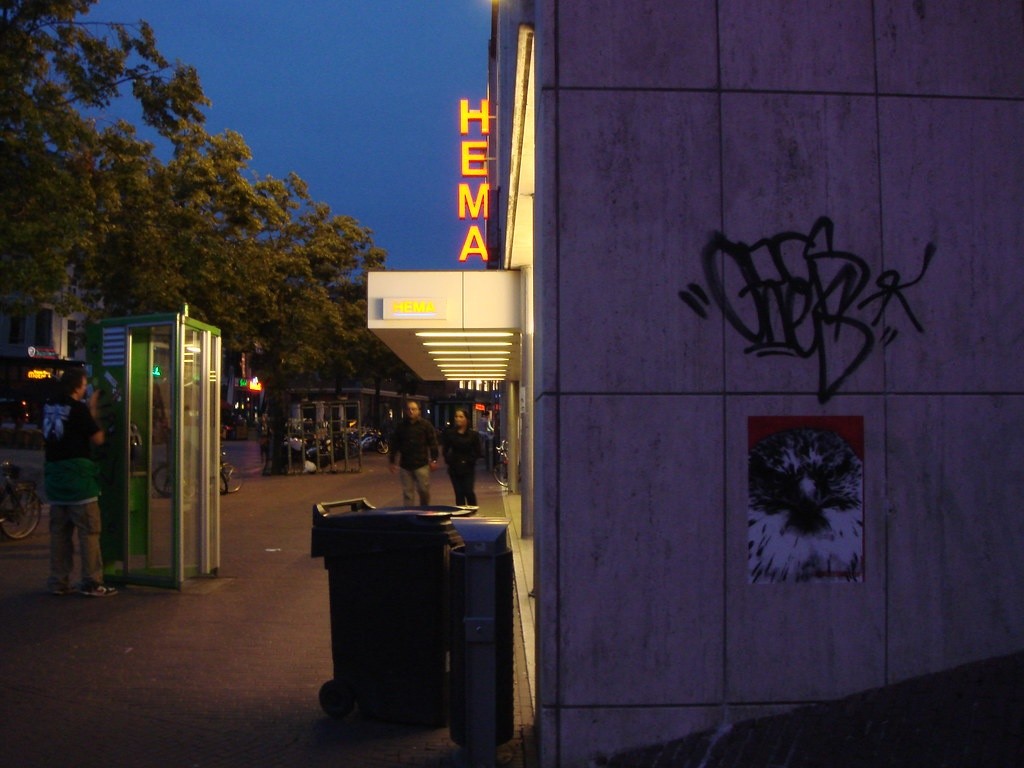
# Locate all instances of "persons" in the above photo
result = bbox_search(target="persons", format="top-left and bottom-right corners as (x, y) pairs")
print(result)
(43, 366), (119, 596)
(442, 407), (484, 512)
(388, 400), (440, 507)
(258, 414), (273, 464)
(480, 410), (495, 433)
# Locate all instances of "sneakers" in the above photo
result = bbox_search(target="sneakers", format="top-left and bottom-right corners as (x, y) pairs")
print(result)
(79, 583), (119, 597)
(51, 585), (77, 595)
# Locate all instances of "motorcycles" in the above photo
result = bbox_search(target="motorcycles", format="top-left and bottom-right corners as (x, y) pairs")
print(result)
(285, 421), (389, 464)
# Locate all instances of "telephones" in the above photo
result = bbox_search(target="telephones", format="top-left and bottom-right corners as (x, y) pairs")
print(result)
(130, 423), (143, 447)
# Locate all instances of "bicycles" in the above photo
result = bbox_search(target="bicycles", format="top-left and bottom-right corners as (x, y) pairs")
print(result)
(493, 439), (510, 487)
(153, 449), (244, 498)
(0, 460), (42, 541)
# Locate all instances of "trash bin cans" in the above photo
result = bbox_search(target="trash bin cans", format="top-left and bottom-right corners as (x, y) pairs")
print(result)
(312, 497), (480, 718)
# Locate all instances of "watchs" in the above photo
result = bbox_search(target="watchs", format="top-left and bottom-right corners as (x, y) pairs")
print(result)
(433, 460), (436, 463)
